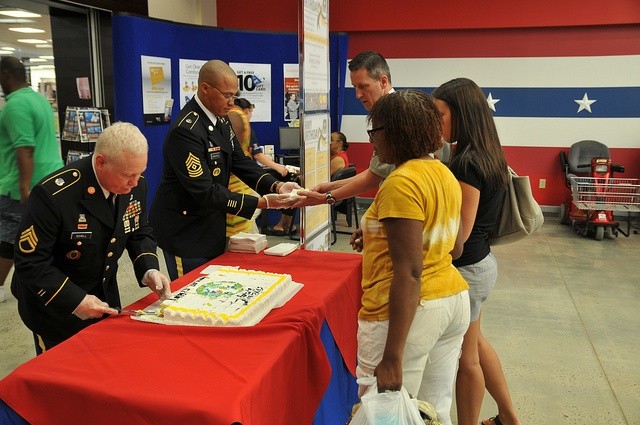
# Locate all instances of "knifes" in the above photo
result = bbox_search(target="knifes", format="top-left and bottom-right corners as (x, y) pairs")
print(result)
(112, 306), (157, 314)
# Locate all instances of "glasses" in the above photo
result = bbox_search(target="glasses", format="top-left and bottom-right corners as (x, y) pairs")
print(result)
(367, 126), (384, 141)
(207, 81), (240, 100)
(331, 137), (340, 142)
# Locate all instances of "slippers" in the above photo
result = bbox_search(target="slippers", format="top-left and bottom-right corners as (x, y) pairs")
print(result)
(262, 224), (285, 235)
(284, 224), (297, 235)
(481, 413), (504, 424)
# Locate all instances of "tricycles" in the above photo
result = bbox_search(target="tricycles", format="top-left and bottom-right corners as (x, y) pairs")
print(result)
(559, 140), (640, 241)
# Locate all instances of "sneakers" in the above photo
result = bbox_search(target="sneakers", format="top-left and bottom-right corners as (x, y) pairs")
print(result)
(0, 285), (6, 303)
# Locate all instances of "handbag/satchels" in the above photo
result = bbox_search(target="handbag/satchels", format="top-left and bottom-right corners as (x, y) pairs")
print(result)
(345, 395), (441, 425)
(487, 163), (546, 246)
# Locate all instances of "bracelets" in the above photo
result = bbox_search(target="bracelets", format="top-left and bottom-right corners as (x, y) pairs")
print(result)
(272, 180), (280, 193)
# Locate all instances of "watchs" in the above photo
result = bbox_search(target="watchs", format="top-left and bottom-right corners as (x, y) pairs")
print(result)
(324, 190), (336, 207)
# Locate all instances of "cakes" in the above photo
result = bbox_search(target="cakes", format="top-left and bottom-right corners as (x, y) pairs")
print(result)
(159, 267), (292, 326)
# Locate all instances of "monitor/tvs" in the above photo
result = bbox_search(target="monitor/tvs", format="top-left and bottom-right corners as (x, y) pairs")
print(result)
(278, 127), (300, 156)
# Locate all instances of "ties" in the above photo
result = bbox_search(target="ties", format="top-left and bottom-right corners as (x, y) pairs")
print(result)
(106, 191), (116, 220)
(215, 117), (222, 136)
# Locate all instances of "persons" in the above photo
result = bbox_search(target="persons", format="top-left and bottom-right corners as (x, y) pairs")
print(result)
(234, 96), (289, 177)
(0, 54), (64, 304)
(328, 131), (349, 176)
(430, 81), (526, 425)
(10, 123), (172, 356)
(290, 50), (452, 208)
(225, 107), (258, 240)
(286, 94), (300, 119)
(148, 60), (306, 284)
(351, 92), (470, 425)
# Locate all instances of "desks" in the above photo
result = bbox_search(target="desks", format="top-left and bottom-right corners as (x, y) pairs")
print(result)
(0, 244), (364, 425)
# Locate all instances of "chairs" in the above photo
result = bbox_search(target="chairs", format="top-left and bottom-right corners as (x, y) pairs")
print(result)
(565, 140), (610, 183)
(289, 194), (337, 244)
(332, 167), (359, 245)
